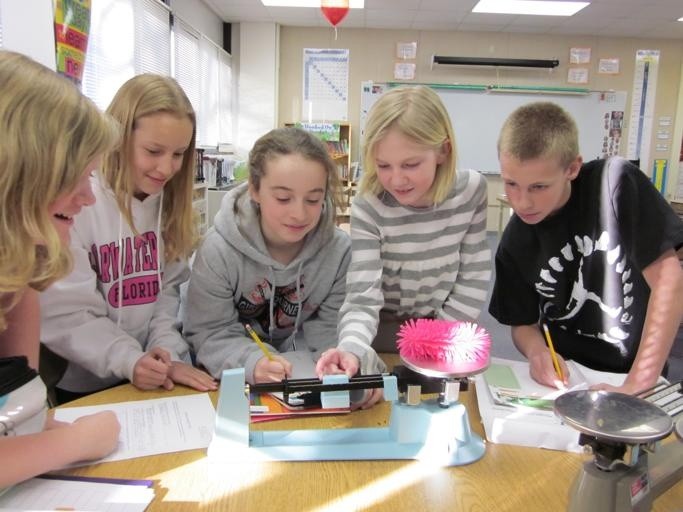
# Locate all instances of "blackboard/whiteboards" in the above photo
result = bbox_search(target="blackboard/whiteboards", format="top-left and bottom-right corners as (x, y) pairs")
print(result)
(360, 81), (628, 176)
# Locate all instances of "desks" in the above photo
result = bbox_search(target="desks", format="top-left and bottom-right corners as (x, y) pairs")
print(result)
(39, 352), (683, 512)
(495, 194), (514, 237)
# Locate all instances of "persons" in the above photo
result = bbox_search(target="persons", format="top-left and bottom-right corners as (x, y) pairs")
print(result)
(488, 102), (682, 394)
(39, 72), (219, 390)
(182, 127), (380, 410)
(316, 83), (493, 379)
(1, 50), (120, 489)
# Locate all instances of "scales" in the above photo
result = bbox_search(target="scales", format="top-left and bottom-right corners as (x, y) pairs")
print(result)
(553, 388), (683, 512)
(206, 318), (494, 470)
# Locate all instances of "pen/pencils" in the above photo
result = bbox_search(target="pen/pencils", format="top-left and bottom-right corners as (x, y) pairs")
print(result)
(543, 323), (563, 380)
(245, 323), (274, 361)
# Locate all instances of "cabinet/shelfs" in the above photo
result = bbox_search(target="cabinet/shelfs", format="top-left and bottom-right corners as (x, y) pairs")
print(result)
(284, 121), (352, 205)
(192, 148), (212, 235)
(205, 154), (240, 226)
